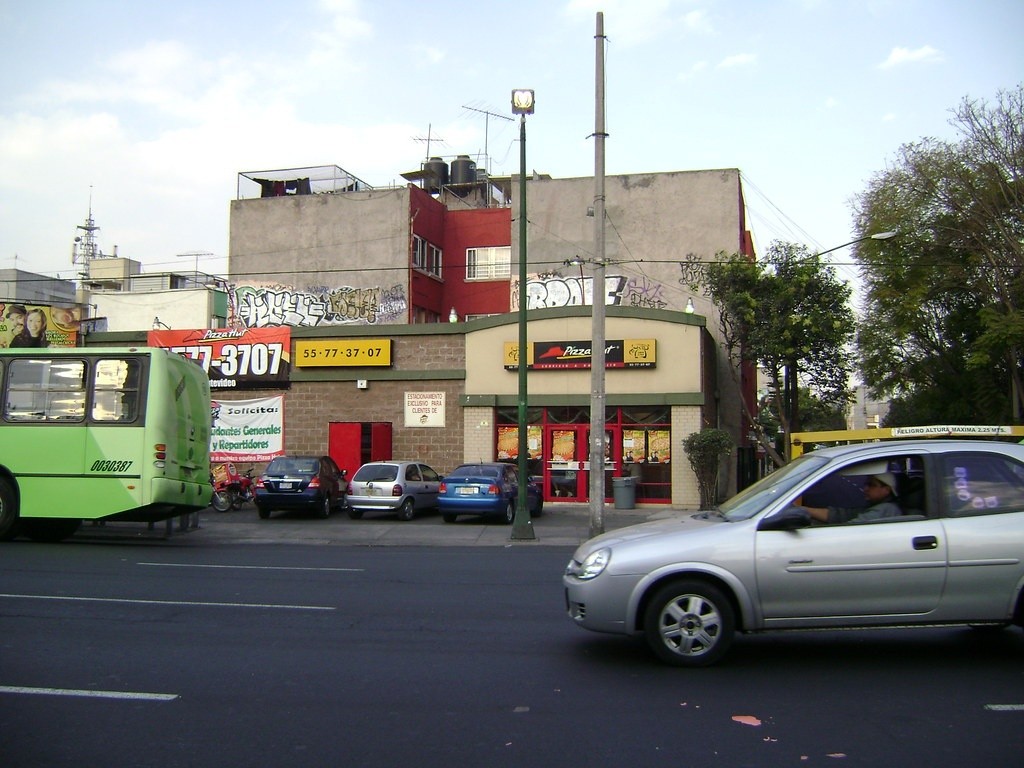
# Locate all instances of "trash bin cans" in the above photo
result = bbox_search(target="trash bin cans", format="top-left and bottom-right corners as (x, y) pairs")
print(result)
(612, 477), (637, 509)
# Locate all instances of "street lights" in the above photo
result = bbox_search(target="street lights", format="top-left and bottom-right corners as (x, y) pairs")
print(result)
(510, 87), (538, 540)
(782, 231), (900, 422)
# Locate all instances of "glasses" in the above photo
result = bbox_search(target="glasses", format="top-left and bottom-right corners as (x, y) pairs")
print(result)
(864, 480), (882, 488)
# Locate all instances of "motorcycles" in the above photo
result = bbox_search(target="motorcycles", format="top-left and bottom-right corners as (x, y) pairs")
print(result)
(226, 466), (259, 511)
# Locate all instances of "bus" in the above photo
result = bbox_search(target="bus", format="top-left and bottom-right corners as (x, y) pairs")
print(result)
(0, 345), (213, 539)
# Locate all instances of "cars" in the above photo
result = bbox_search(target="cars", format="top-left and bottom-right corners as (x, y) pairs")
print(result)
(252, 455), (350, 519)
(340, 459), (443, 520)
(437, 460), (544, 523)
(562, 437), (1024, 668)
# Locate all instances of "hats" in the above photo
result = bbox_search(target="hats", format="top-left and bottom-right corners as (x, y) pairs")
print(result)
(874, 472), (897, 497)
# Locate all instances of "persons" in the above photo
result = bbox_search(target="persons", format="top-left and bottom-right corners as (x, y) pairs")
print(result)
(792, 470), (902, 523)
(626, 451), (633, 461)
(5, 304), (48, 348)
(651, 451), (659, 462)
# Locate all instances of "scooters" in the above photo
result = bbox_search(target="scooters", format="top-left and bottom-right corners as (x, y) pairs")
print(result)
(207, 459), (233, 513)
